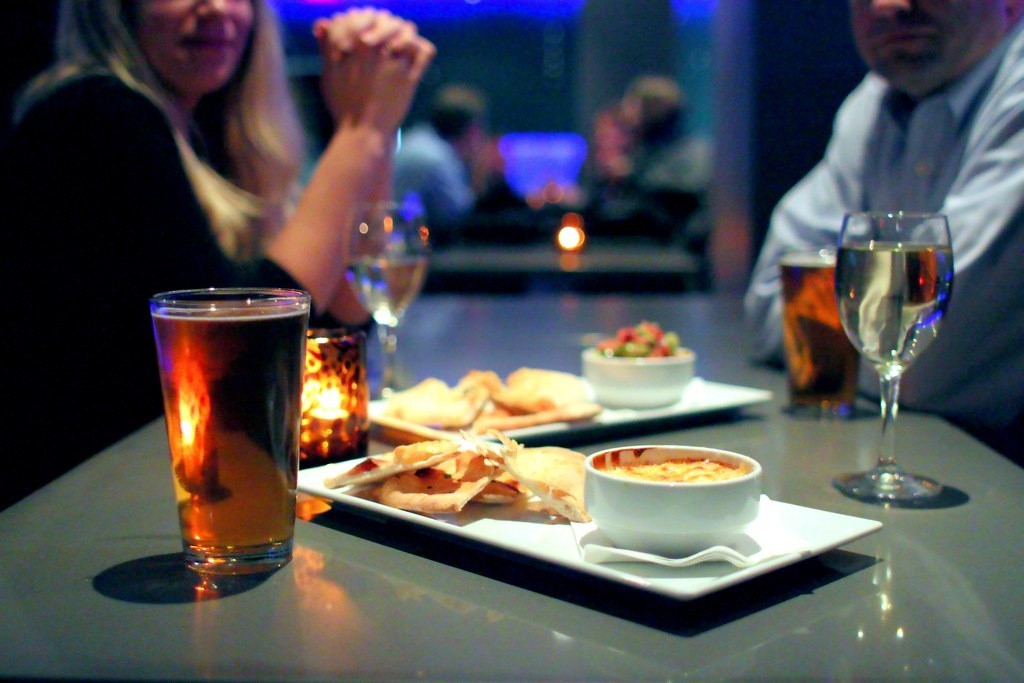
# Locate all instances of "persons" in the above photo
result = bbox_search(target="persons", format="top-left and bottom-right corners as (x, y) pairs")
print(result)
(425, 77), (715, 245)
(746, 1), (1024, 433)
(0, 0), (435, 503)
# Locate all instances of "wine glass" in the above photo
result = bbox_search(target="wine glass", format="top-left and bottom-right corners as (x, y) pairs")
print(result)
(342, 199), (427, 401)
(833, 211), (954, 502)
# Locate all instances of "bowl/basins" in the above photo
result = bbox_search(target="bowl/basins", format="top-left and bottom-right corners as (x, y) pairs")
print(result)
(583, 348), (696, 410)
(583, 446), (763, 555)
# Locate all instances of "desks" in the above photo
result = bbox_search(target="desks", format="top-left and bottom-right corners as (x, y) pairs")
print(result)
(0, 296), (1024, 683)
(436, 240), (706, 295)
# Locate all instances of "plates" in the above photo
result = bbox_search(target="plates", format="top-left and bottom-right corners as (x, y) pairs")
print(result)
(366, 378), (774, 442)
(297, 443), (884, 600)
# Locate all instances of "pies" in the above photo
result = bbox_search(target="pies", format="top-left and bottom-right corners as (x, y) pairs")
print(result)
(324, 367), (601, 521)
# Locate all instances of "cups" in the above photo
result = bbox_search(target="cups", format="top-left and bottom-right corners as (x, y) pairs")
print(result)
(296, 324), (370, 464)
(151, 287), (312, 576)
(778, 247), (861, 417)
